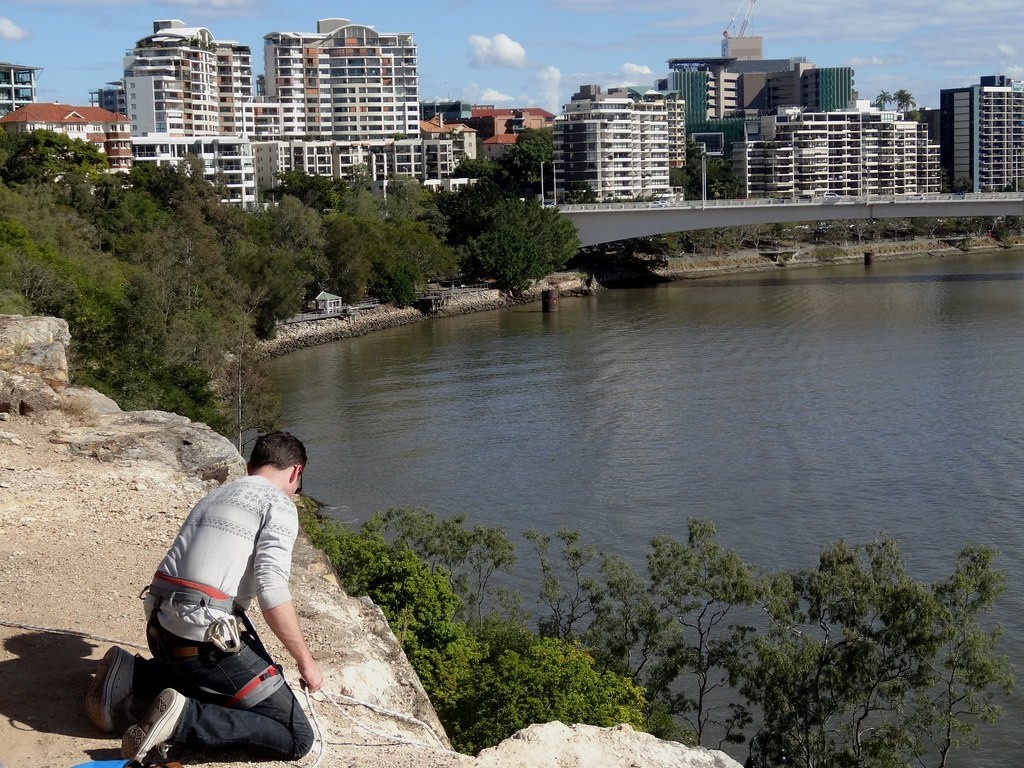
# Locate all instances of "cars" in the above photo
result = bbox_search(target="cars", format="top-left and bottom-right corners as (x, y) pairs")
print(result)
(949, 192), (966, 200)
(906, 192), (925, 201)
(767, 196), (793, 205)
(647, 200), (670, 207)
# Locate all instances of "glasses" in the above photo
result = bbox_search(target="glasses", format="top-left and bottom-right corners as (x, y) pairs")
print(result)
(292, 465), (302, 494)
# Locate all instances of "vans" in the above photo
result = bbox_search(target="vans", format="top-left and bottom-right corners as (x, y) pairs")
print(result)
(824, 193), (842, 199)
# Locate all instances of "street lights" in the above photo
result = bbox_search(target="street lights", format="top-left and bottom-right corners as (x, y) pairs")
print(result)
(552, 162), (557, 207)
(541, 161), (545, 206)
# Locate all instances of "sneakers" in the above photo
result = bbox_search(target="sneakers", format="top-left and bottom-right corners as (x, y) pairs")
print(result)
(84, 645), (134, 734)
(120, 687), (189, 764)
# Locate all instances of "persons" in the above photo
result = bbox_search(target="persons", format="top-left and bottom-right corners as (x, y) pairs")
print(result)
(84, 432), (323, 766)
